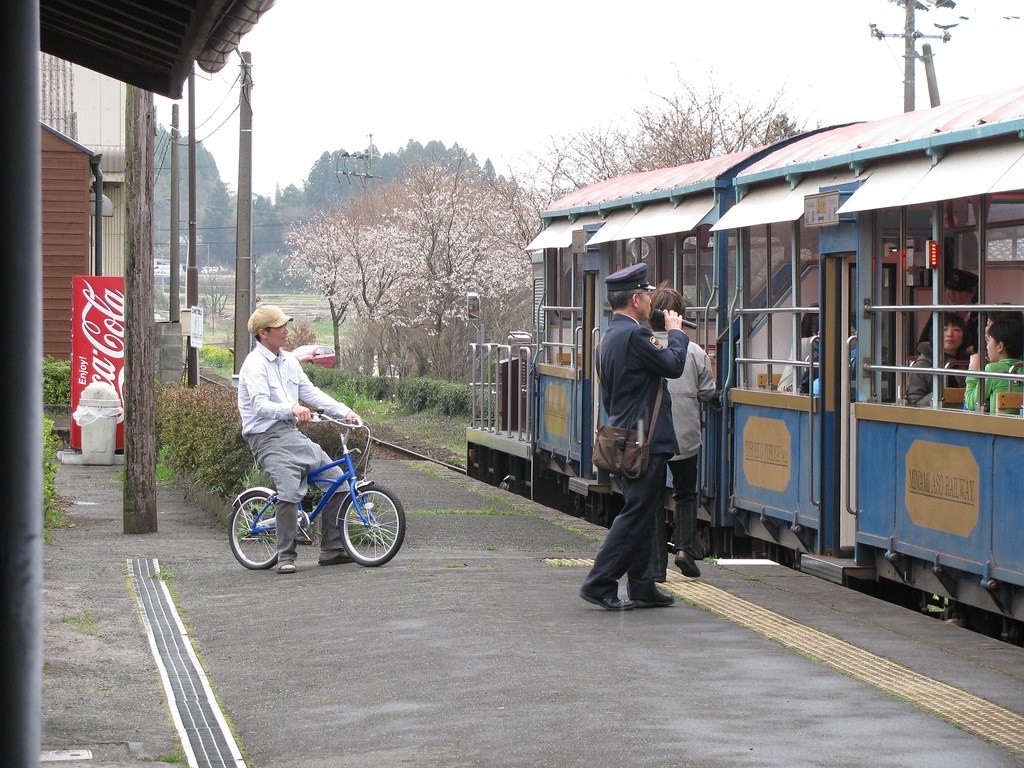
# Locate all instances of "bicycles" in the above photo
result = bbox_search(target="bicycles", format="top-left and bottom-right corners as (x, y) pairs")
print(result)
(225, 407), (407, 569)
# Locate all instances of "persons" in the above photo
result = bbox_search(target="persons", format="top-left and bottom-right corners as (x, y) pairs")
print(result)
(578, 262), (690, 611)
(800, 314), (819, 393)
(776, 303), (819, 394)
(906, 313), (970, 408)
(985, 303), (1024, 335)
(850, 319), (858, 380)
(647, 279), (718, 584)
(916, 268), (988, 388)
(237, 305), (364, 574)
(964, 320), (1024, 415)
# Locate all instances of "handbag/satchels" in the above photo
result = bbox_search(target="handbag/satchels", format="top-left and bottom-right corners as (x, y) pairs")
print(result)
(592, 426), (650, 478)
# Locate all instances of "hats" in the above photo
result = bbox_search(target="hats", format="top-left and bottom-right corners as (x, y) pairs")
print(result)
(944, 267), (978, 291)
(247, 306), (293, 337)
(603, 263), (658, 292)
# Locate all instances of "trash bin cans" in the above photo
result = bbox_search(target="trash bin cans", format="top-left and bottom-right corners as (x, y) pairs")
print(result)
(71, 381), (124, 466)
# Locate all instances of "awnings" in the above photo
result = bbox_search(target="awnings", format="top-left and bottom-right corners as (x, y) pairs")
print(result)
(584, 192), (715, 245)
(834, 139), (1024, 213)
(524, 213), (602, 250)
(709, 169), (855, 232)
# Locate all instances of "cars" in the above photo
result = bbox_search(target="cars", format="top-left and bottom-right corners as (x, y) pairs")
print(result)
(291, 344), (336, 368)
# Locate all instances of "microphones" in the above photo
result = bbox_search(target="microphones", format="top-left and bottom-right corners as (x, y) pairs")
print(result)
(653, 308), (698, 330)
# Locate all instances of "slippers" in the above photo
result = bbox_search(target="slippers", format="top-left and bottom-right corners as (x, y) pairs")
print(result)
(318, 551), (356, 565)
(275, 560), (296, 574)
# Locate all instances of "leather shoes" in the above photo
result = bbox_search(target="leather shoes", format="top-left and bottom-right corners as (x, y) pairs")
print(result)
(654, 574), (666, 581)
(675, 551), (701, 578)
(633, 590), (675, 606)
(579, 589), (636, 610)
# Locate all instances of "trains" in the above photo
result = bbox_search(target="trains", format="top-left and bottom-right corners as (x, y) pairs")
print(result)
(466, 97), (1024, 645)
